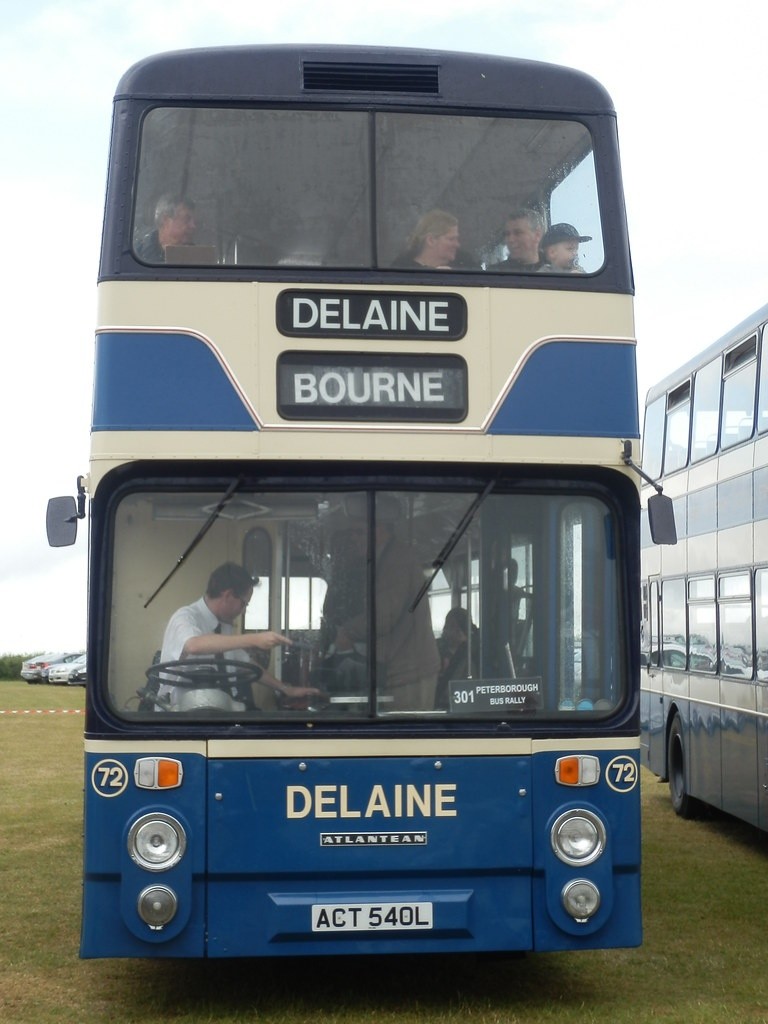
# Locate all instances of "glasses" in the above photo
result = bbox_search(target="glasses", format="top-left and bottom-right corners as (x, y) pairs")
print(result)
(234, 595), (249, 608)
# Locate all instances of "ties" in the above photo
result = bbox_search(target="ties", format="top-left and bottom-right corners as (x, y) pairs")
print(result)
(214, 623), (233, 698)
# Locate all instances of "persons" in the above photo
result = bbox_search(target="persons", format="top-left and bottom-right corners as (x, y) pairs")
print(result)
(439, 608), (473, 668)
(336, 517), (441, 711)
(543, 224), (594, 273)
(153, 563), (319, 712)
(490, 208), (550, 273)
(137, 194), (197, 263)
(394, 210), (461, 271)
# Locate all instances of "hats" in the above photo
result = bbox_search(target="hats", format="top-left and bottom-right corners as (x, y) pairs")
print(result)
(539, 223), (592, 247)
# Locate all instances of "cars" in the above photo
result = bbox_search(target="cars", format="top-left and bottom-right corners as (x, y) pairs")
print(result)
(20, 652), (69, 684)
(49, 652), (87, 685)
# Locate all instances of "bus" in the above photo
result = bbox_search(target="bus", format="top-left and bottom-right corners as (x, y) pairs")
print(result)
(640, 300), (767, 837)
(45, 42), (679, 962)
